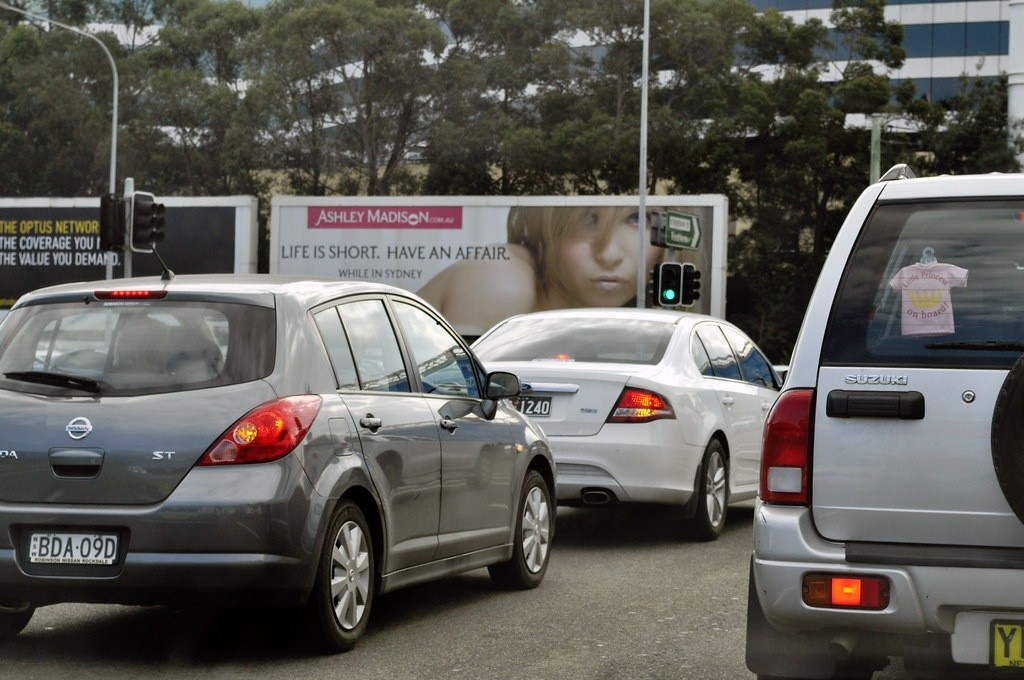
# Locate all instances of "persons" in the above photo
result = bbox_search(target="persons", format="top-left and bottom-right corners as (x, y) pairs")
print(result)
(414, 205), (708, 336)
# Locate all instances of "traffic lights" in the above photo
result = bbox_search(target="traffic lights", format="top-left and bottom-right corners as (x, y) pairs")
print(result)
(681, 263), (701, 307)
(100, 192), (126, 252)
(130, 191), (166, 253)
(658, 262), (681, 307)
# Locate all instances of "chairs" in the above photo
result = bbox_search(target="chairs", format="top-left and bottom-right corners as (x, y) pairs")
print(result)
(547, 339), (598, 358)
(166, 338), (224, 373)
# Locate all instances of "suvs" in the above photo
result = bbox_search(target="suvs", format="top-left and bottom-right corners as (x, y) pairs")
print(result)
(745, 162), (1024, 680)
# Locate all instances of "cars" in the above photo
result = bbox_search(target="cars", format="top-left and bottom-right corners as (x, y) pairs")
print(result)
(0, 274), (557, 650)
(467, 308), (788, 541)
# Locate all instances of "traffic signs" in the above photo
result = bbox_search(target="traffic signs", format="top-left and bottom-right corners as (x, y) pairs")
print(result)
(664, 211), (702, 250)
